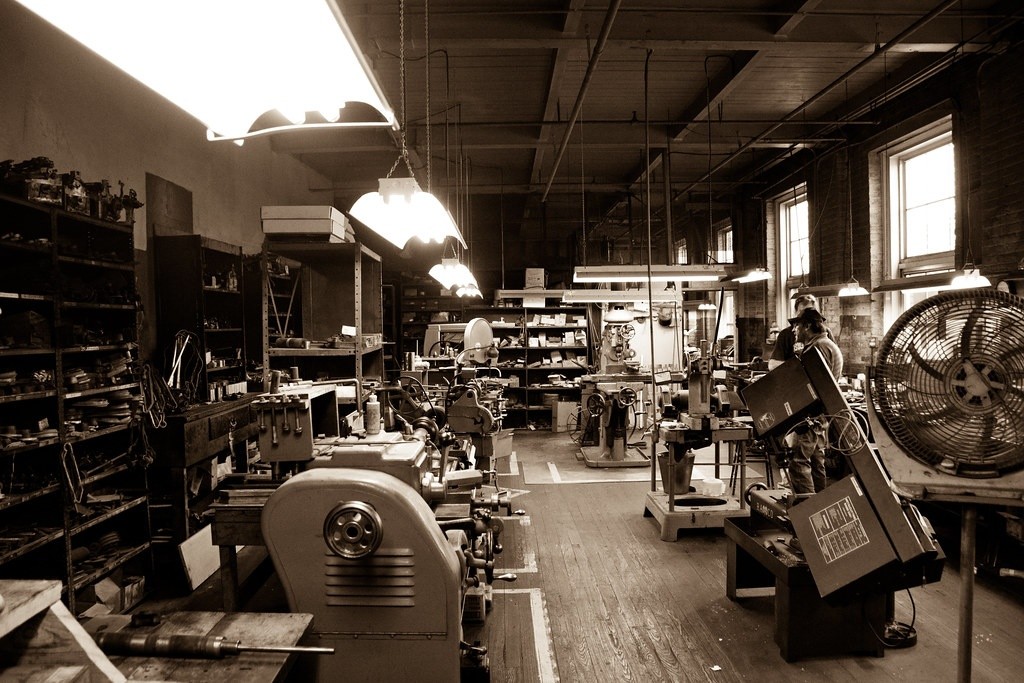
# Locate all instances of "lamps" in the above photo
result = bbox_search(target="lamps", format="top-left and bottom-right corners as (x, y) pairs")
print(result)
(15, 0), (484, 299)
(789, 78), (870, 299)
(871, 13), (992, 293)
(493, 107), (717, 305)
(717, 151), (774, 284)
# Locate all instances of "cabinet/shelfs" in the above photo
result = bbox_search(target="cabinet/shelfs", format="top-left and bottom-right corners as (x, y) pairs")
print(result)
(1, 159), (590, 623)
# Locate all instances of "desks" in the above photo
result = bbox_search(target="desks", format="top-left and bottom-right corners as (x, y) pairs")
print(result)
(145, 392), (270, 541)
(0, 579), (315, 682)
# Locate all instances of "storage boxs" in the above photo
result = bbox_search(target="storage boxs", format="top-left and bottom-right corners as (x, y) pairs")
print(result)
(75, 565), (146, 616)
(577, 356), (586, 367)
(552, 401), (579, 433)
(260, 206), (345, 227)
(540, 393), (559, 406)
(261, 220), (345, 241)
(548, 373), (566, 384)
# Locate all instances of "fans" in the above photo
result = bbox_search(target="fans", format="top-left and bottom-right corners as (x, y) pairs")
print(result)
(863, 287), (1023, 683)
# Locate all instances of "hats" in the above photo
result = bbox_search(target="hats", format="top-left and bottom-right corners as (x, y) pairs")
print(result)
(787, 307), (826, 323)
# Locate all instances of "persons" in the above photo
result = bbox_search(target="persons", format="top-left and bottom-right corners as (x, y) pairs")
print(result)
(769, 295), (836, 481)
(788, 308), (844, 505)
